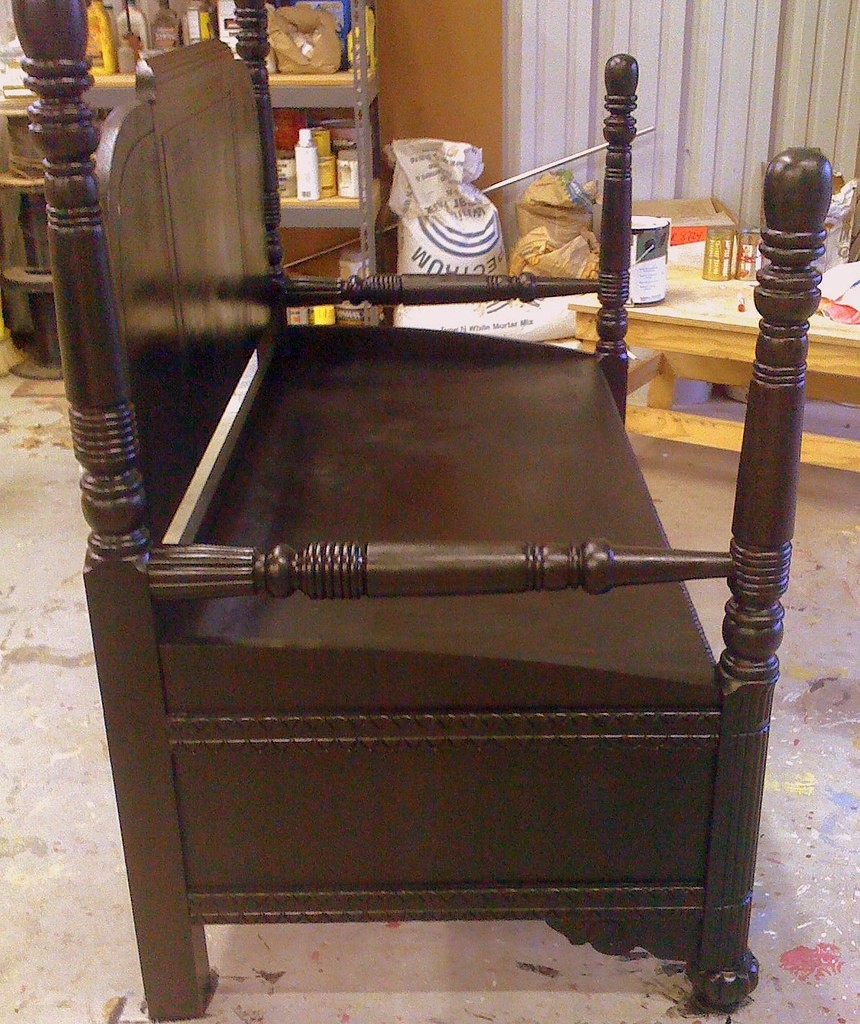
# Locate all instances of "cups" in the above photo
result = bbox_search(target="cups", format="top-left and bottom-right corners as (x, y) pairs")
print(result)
(735, 232), (764, 281)
(702, 225), (735, 281)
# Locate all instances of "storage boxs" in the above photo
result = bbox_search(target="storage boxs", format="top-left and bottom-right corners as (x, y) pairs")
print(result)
(631, 195), (739, 247)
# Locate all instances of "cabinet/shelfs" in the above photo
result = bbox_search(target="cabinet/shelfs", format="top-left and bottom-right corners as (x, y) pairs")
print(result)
(0, 0), (391, 327)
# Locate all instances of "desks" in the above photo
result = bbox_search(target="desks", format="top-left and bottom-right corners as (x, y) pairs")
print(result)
(568, 266), (860, 477)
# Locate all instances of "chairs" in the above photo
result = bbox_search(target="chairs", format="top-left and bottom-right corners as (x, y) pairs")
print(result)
(8, 0), (834, 1022)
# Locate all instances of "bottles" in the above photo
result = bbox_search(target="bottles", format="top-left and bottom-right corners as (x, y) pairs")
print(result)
(294, 128), (320, 201)
(336, 149), (359, 199)
(311, 129), (338, 199)
(275, 150), (297, 198)
(86, 0), (181, 76)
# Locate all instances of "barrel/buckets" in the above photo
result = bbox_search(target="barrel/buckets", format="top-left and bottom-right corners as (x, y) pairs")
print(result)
(628, 216), (671, 309)
